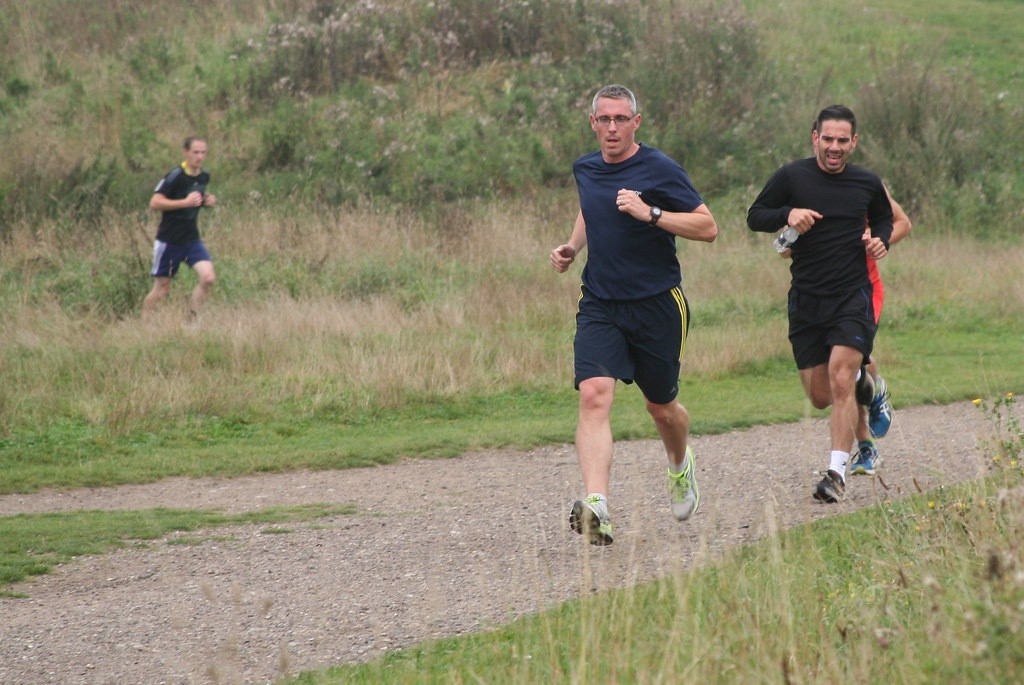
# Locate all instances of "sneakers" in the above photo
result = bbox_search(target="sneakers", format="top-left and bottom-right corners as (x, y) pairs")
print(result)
(569, 498), (613, 546)
(667, 444), (701, 522)
(849, 439), (882, 475)
(867, 375), (893, 439)
(855, 364), (875, 406)
(812, 470), (845, 503)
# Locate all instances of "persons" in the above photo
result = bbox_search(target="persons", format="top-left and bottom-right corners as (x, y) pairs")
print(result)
(544, 83), (722, 548)
(776, 115), (914, 479)
(742, 102), (898, 508)
(140, 133), (219, 330)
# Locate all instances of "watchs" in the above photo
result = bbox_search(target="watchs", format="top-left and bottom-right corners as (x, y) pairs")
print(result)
(646, 206), (662, 227)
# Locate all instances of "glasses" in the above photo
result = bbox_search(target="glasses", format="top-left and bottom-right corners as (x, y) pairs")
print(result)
(595, 114), (635, 126)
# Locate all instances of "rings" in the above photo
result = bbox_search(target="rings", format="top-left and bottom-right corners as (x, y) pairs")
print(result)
(619, 199), (623, 206)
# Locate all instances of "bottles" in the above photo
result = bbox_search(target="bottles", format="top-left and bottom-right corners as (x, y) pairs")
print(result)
(772, 226), (799, 254)
